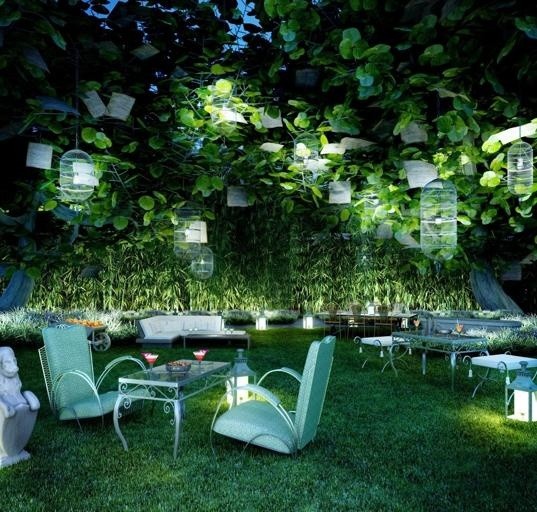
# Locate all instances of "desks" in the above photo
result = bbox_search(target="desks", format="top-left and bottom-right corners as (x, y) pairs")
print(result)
(111, 357), (232, 460)
(314, 310), (420, 337)
(391, 329), (488, 394)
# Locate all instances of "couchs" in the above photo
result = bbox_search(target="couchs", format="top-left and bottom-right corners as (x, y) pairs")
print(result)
(134, 309), (224, 350)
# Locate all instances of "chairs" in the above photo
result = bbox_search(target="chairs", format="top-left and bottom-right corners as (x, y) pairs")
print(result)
(34, 323), (156, 435)
(322, 298), (404, 342)
(207, 334), (337, 462)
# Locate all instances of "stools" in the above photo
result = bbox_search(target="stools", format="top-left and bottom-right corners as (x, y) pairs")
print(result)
(355, 334), (412, 379)
(461, 351), (537, 402)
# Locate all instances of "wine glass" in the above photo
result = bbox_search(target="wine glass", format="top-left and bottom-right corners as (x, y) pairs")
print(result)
(456, 324), (463, 338)
(414, 320), (420, 331)
(193, 350), (206, 369)
(144, 354), (158, 376)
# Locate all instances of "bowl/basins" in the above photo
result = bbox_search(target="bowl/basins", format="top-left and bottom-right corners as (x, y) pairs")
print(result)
(440, 330), (452, 334)
(165, 365), (191, 372)
